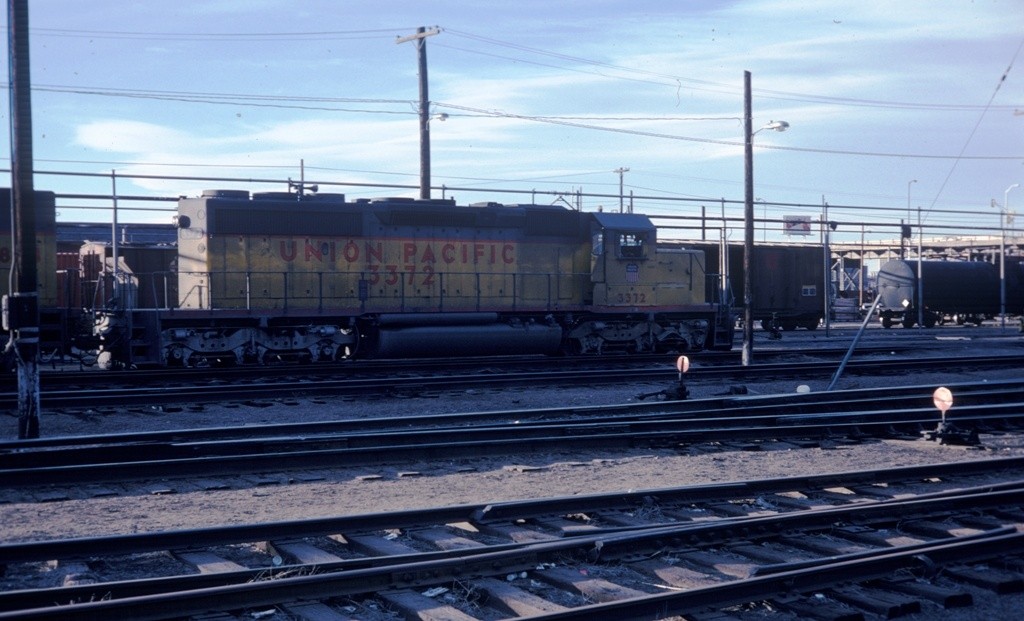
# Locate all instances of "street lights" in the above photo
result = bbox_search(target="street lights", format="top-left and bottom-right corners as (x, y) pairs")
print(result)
(1001, 181), (1024, 235)
(419, 111), (450, 201)
(753, 196), (768, 243)
(738, 69), (788, 369)
(907, 178), (919, 232)
(611, 165), (632, 212)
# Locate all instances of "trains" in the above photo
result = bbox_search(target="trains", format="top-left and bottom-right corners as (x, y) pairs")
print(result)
(874, 251), (1024, 329)
(659, 235), (834, 334)
(0, 182), (741, 387)
